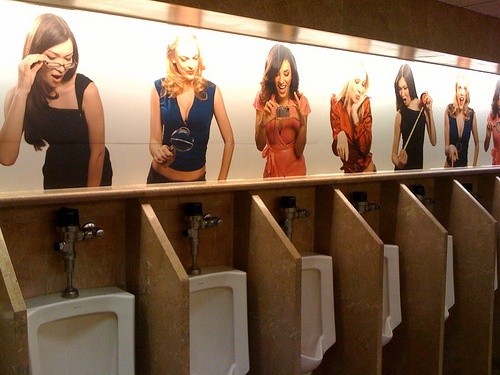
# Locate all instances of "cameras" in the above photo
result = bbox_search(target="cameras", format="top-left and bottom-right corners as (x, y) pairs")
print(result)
(276, 106), (289, 118)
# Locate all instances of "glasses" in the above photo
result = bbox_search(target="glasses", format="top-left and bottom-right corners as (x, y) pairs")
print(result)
(44, 60), (77, 70)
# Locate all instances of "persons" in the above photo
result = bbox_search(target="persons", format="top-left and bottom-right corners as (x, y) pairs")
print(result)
(484, 80), (500, 167)
(0, 14), (112, 190)
(253, 43), (311, 178)
(391, 64), (436, 171)
(147, 32), (235, 183)
(444, 78), (480, 167)
(329, 70), (377, 173)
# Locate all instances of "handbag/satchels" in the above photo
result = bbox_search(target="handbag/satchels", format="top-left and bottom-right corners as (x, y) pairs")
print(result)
(397, 105), (423, 170)
